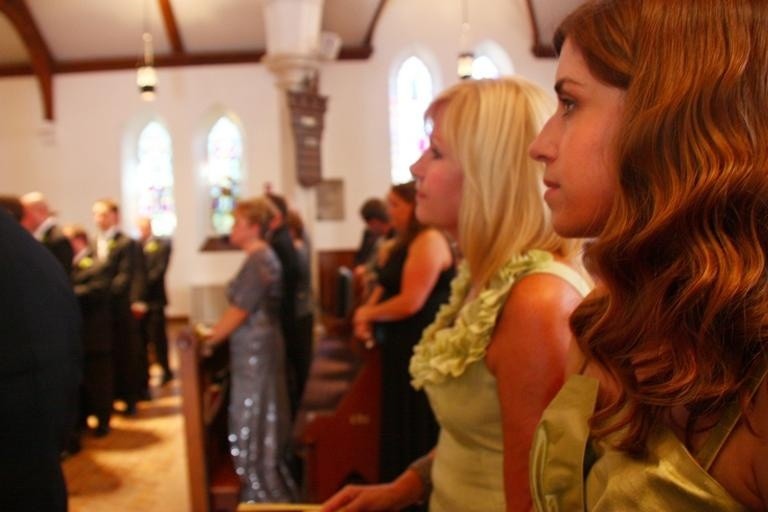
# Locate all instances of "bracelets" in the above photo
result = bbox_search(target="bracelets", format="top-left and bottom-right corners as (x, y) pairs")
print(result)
(408, 456), (433, 503)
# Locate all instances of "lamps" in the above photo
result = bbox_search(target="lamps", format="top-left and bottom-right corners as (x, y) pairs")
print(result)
(457, 1), (475, 81)
(136, 0), (158, 102)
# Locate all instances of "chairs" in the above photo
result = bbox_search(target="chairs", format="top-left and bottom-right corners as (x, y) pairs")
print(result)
(296, 267), (377, 504)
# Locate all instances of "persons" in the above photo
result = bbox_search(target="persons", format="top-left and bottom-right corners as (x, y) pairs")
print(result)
(207, 196), (297, 506)
(23, 189), (174, 456)
(318, 73), (589, 512)
(258, 192), (308, 422)
(288, 211), (313, 401)
(527, 0), (767, 512)
(1, 200), (89, 512)
(352, 179), (453, 479)
